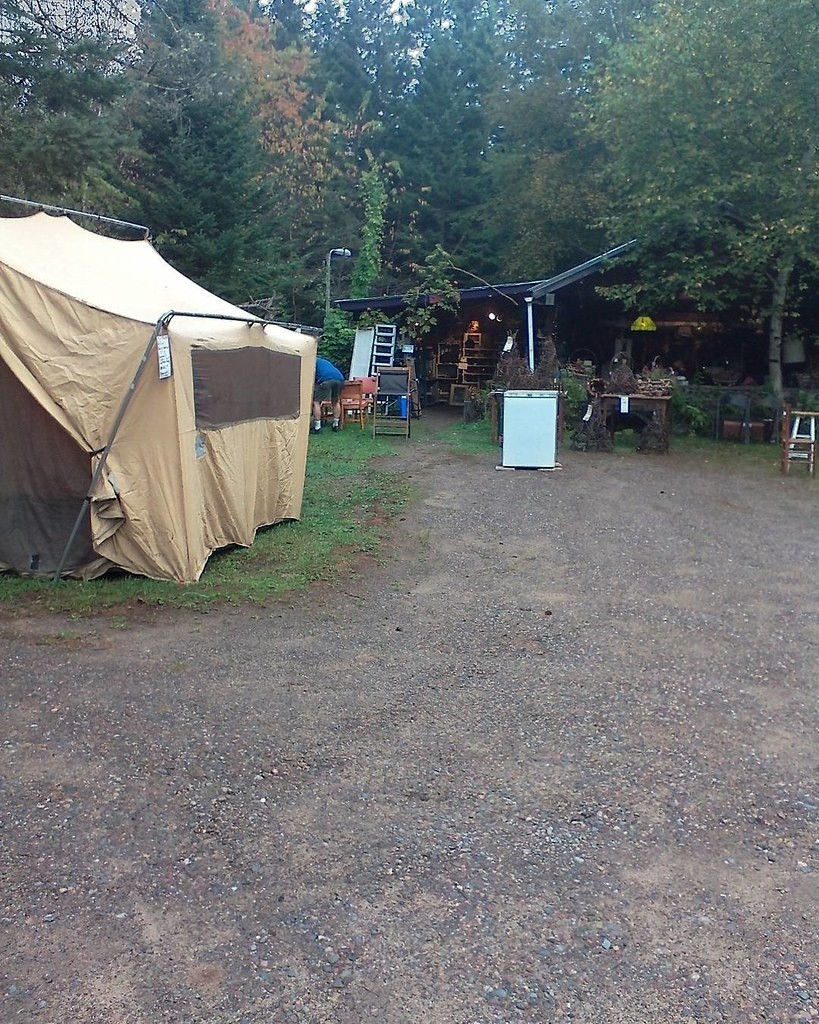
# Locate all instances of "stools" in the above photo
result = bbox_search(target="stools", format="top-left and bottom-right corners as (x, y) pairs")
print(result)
(784, 411), (819, 472)
(322, 376), (377, 428)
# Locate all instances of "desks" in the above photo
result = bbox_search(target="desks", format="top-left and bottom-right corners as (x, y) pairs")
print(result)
(599, 393), (672, 441)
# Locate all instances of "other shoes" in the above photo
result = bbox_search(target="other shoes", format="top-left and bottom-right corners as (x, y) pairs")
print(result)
(332, 426), (338, 432)
(309, 427), (322, 434)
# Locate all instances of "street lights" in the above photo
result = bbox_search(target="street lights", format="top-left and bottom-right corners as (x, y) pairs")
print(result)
(324, 245), (352, 317)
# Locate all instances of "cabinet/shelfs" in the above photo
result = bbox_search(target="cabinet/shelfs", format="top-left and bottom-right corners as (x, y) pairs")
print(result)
(419, 333), (501, 405)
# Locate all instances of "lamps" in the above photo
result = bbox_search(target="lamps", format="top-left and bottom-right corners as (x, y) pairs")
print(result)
(631, 316), (656, 331)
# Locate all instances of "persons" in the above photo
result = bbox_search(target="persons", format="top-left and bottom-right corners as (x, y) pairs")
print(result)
(310, 358), (345, 435)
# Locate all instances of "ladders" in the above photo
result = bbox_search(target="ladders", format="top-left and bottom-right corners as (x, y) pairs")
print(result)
(368, 324), (397, 415)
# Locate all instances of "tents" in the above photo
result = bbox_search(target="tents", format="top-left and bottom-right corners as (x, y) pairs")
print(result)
(0, 195), (324, 586)
